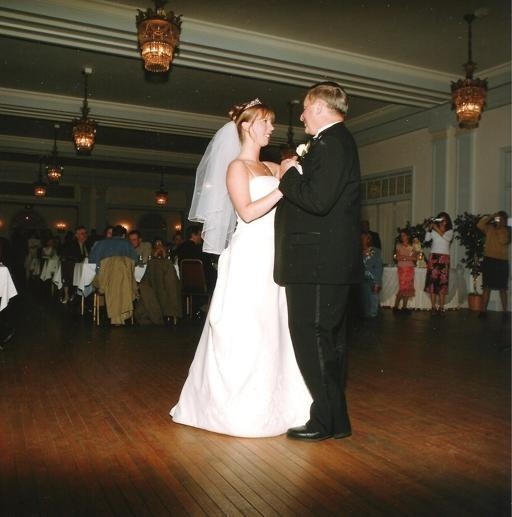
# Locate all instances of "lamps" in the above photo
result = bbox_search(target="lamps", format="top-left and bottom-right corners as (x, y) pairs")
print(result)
(134, 1), (186, 74)
(34, 156), (47, 198)
(74, 66), (100, 155)
(155, 165), (170, 203)
(45, 128), (64, 179)
(447, 13), (487, 129)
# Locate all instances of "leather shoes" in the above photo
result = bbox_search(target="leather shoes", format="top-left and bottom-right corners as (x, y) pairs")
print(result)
(285, 418), (353, 442)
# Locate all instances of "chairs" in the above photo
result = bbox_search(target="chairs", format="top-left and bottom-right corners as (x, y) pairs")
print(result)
(24, 232), (218, 329)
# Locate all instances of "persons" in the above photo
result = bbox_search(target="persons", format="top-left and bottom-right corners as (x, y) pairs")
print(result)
(475, 211), (512, 322)
(394, 229), (417, 314)
(271, 80), (365, 441)
(424, 212), (455, 314)
(362, 234), (383, 319)
(362, 220), (381, 248)
(39, 222), (219, 325)
(169, 98), (314, 437)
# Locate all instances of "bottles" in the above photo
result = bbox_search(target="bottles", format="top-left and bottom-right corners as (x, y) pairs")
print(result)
(149, 255), (172, 261)
(138, 252), (144, 264)
(417, 249), (424, 260)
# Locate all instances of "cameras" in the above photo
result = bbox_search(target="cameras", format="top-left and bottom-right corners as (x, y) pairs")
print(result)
(494, 217), (500, 222)
(430, 217), (443, 223)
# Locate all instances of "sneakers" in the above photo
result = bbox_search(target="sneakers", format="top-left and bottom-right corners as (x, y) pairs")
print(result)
(392, 305), (509, 322)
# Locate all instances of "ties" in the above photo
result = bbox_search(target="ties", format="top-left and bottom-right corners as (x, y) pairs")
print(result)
(82, 244), (86, 258)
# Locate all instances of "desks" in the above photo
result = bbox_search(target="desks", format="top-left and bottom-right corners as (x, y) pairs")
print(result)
(0, 262), (18, 311)
(376, 265), (465, 312)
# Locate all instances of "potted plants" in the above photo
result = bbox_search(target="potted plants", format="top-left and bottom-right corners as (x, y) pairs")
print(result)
(452, 210), (496, 312)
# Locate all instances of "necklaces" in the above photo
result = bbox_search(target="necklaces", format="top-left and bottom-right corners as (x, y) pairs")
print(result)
(258, 167), (269, 176)
